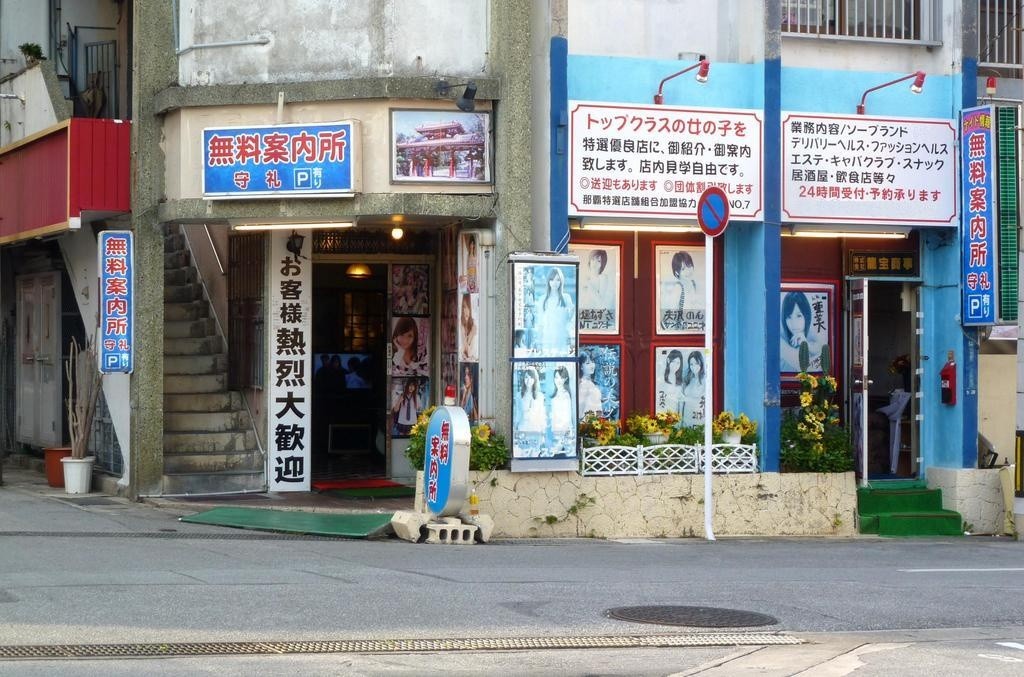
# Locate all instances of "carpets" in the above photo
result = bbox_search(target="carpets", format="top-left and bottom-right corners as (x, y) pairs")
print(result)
(312, 478), (404, 491)
(331, 486), (416, 498)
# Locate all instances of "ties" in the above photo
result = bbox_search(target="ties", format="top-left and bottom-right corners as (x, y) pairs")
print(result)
(406, 394), (412, 422)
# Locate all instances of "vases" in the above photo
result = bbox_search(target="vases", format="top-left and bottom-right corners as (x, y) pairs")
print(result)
(584, 437), (600, 447)
(724, 430), (741, 445)
(42, 447), (72, 487)
(60, 457), (94, 494)
(643, 432), (669, 445)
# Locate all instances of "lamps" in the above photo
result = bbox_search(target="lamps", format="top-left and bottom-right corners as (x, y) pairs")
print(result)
(345, 263), (371, 280)
(287, 236), (304, 254)
(857, 70), (925, 115)
(655, 60), (710, 104)
(437, 80), (476, 113)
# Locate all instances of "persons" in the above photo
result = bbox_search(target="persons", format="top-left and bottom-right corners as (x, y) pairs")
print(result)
(660, 251), (705, 329)
(514, 266), (576, 355)
(466, 234), (480, 294)
(396, 269), (423, 314)
(459, 363), (479, 426)
(576, 348), (603, 420)
(579, 249), (615, 310)
(656, 349), (705, 426)
(513, 366), (575, 447)
(393, 377), (425, 435)
(315, 355), (382, 397)
(392, 317), (429, 377)
(779, 290), (824, 373)
(458, 292), (478, 361)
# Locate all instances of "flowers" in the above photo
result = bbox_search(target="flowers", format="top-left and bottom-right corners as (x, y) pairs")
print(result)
(630, 414), (684, 439)
(578, 413), (622, 443)
(712, 412), (755, 439)
